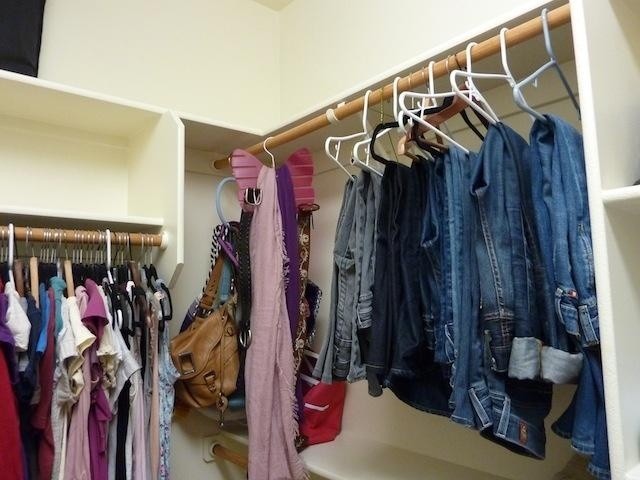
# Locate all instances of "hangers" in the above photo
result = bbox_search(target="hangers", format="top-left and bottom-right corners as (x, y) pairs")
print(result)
(216, 135), (296, 231)
(1, 223), (158, 275)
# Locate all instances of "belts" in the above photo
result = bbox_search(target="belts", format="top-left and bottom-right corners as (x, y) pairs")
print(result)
(235, 187), (263, 349)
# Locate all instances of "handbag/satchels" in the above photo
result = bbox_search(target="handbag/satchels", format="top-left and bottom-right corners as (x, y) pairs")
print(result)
(162, 303), (245, 411)
(295, 351), (347, 447)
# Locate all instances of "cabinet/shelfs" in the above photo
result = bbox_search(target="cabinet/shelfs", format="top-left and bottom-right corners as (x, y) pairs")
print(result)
(0, 70), (184, 289)
